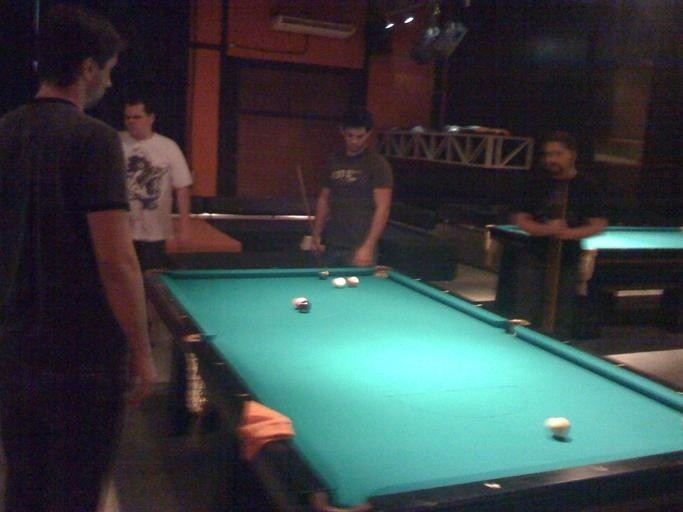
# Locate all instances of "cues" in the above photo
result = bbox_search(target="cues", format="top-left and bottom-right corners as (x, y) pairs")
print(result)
(540, 173), (571, 335)
(294, 160), (324, 267)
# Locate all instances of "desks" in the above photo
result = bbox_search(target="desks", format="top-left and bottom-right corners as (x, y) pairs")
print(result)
(488, 226), (683, 335)
(165, 217), (241, 256)
(147, 264), (681, 510)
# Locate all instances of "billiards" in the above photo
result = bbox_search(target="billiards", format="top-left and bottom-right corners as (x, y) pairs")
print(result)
(544, 416), (571, 437)
(293, 296), (309, 310)
(332, 276), (360, 287)
(318, 269), (330, 279)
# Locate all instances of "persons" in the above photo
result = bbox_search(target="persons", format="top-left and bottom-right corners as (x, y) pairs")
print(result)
(1, 12), (156, 512)
(309, 107), (394, 268)
(509, 130), (608, 340)
(117, 98), (194, 272)
